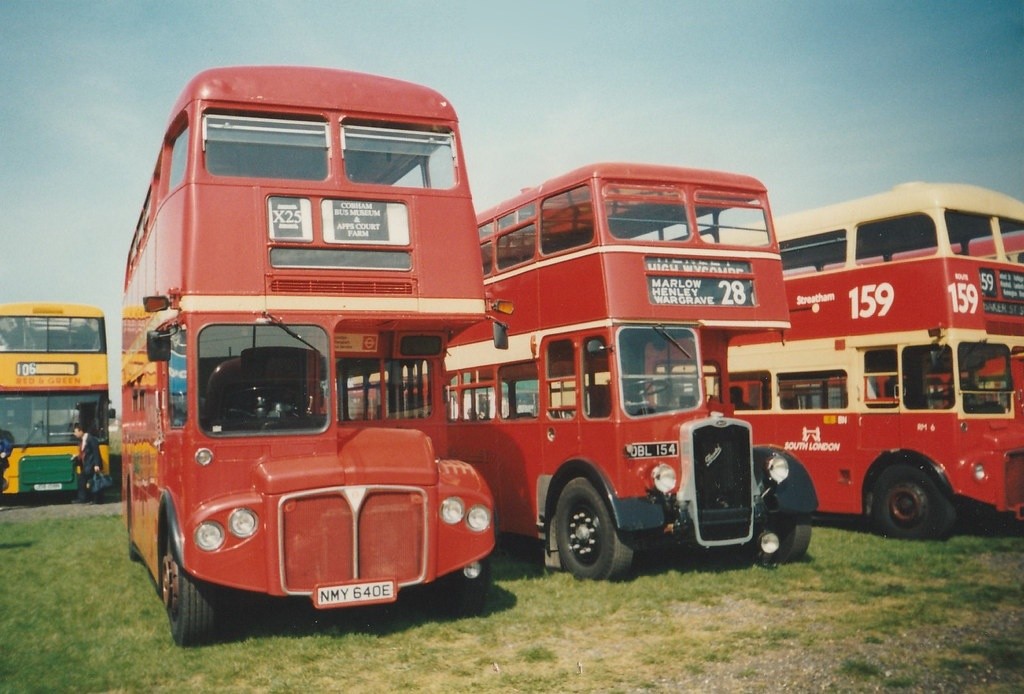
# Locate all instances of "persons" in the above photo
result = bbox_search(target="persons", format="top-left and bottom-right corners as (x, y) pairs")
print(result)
(71, 422), (101, 506)
(0, 438), (12, 492)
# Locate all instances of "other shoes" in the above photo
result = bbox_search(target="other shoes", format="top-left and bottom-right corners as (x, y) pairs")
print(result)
(88, 498), (100, 505)
(72, 499), (86, 504)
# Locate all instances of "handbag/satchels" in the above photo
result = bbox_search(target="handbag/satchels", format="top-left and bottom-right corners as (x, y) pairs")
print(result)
(91, 471), (113, 492)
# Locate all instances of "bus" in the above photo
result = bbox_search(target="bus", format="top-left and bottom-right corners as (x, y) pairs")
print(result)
(0, 301), (116, 507)
(641, 183), (1024, 544)
(347, 162), (820, 583)
(118, 66), (515, 648)
